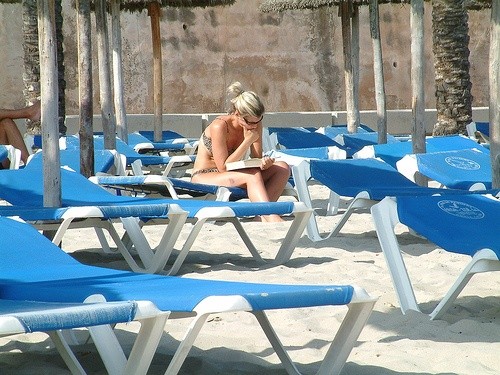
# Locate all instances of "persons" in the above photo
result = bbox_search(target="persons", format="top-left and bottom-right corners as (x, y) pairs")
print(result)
(0, 99), (41, 170)
(190, 81), (291, 222)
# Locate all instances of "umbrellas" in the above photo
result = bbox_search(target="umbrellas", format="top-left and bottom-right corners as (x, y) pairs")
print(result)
(258, 0), (432, 134)
(70, 0), (236, 141)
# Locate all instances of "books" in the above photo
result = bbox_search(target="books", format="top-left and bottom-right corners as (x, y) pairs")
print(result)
(225, 156), (281, 171)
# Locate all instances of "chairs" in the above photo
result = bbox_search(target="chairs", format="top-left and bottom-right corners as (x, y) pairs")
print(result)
(0, 121), (500, 375)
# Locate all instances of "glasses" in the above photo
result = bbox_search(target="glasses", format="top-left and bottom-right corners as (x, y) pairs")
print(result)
(243, 115), (264, 125)
